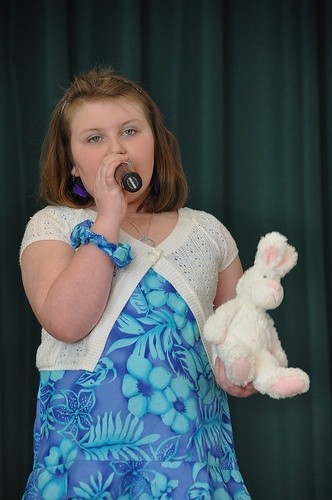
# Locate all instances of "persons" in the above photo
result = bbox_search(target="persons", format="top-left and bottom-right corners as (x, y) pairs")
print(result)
(19, 70), (252, 500)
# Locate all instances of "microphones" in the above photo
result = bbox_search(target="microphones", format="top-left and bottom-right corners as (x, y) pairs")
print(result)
(115, 160), (142, 193)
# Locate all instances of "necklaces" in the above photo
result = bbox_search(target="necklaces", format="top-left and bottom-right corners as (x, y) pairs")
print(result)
(123, 206), (160, 250)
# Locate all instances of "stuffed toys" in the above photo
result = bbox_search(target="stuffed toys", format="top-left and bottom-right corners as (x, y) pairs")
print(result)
(199, 230), (311, 402)
